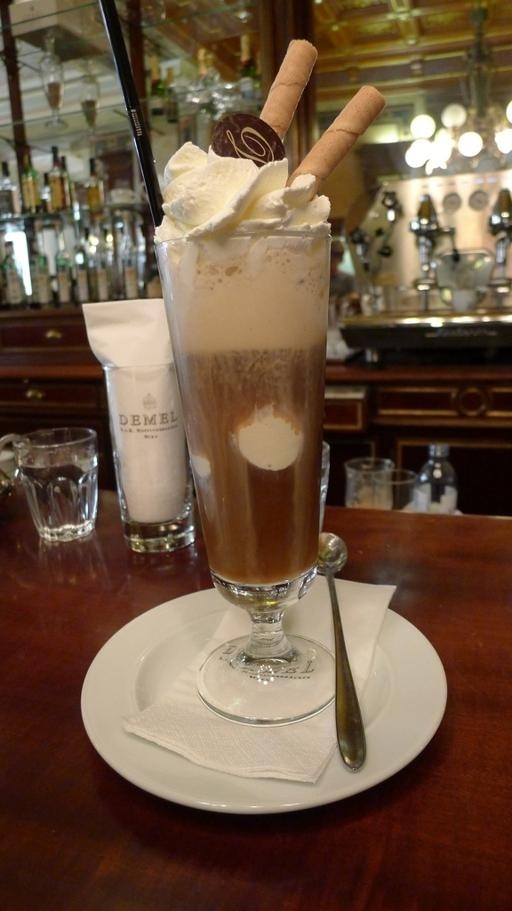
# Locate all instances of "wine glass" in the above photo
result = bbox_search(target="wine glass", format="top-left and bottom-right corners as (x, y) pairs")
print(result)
(156, 228), (330, 729)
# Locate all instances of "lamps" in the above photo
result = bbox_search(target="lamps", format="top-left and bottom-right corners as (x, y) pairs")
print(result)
(76, 58), (99, 133)
(38, 36), (69, 130)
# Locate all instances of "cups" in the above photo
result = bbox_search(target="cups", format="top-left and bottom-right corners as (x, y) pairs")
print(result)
(11, 364), (197, 554)
(343, 456), (415, 514)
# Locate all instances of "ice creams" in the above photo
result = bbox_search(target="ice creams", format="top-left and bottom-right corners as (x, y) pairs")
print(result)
(149, 38), (385, 355)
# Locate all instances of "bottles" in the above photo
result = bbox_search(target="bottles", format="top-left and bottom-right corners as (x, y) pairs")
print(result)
(0, 33), (256, 312)
(415, 443), (458, 514)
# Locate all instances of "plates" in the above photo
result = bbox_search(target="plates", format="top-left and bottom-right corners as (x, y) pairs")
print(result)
(78, 588), (449, 815)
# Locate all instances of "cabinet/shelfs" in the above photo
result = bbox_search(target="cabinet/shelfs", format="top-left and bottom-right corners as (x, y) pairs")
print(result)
(0, 307), (116, 491)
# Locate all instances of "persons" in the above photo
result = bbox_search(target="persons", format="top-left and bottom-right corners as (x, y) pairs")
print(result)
(330, 241), (355, 295)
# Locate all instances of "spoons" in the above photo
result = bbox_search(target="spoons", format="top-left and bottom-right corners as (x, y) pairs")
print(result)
(314, 532), (367, 773)
